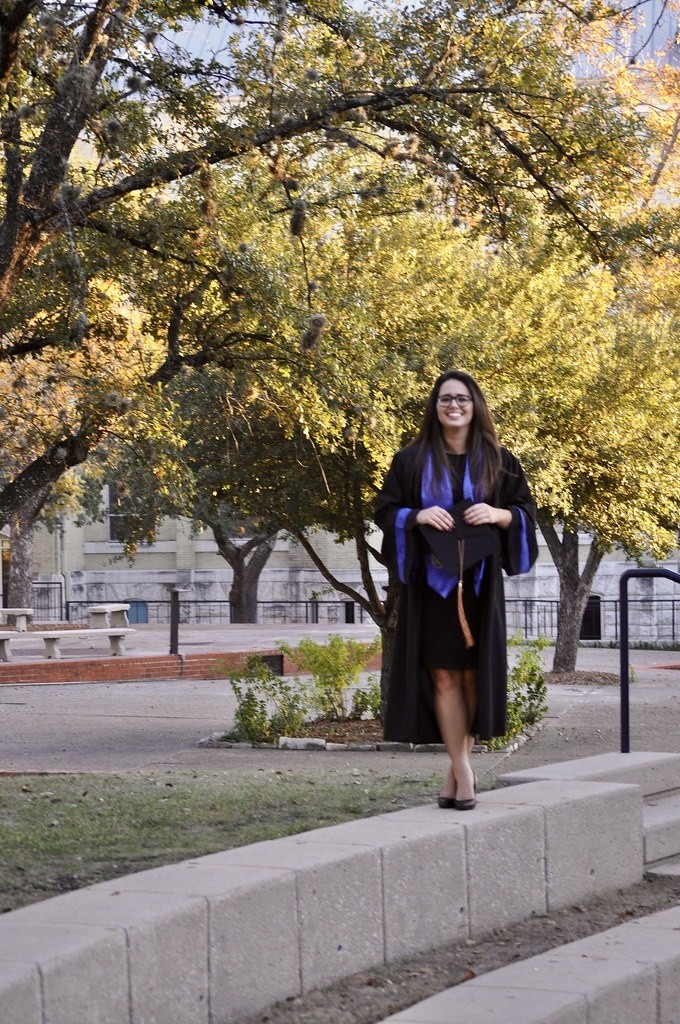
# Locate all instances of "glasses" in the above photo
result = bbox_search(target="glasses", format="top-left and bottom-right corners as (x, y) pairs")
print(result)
(436, 395), (473, 407)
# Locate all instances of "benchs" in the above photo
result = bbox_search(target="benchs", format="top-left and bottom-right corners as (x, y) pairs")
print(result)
(0, 608), (34, 632)
(0, 631), (19, 662)
(87, 604), (131, 629)
(32, 628), (136, 659)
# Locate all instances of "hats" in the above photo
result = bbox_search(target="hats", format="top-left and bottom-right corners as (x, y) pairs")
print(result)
(419, 497), (502, 647)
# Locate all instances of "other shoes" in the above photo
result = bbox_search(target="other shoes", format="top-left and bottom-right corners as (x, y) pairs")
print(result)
(437, 770), (477, 810)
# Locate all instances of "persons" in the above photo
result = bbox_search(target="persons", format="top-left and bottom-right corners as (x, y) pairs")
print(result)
(371, 371), (539, 810)
